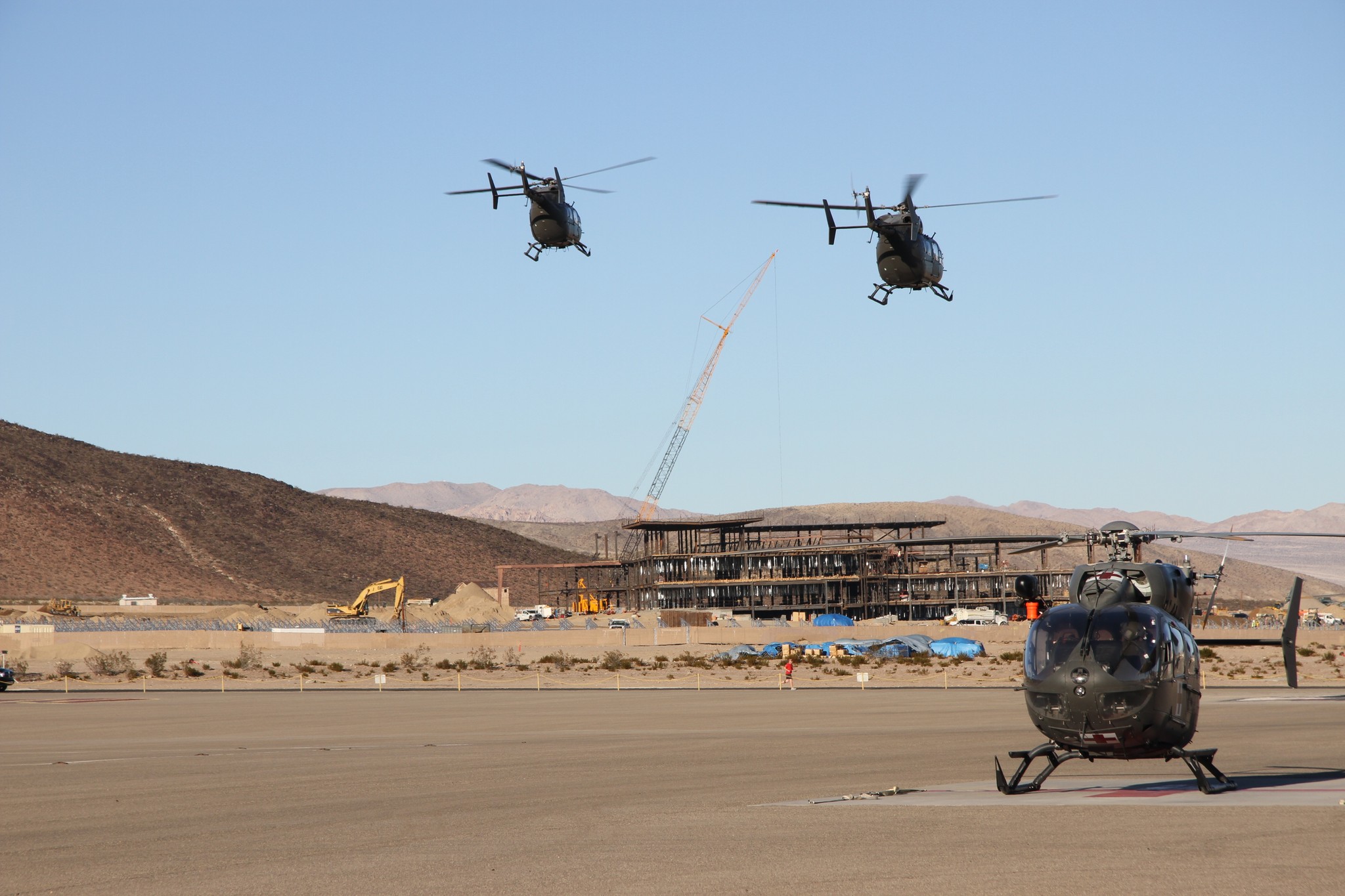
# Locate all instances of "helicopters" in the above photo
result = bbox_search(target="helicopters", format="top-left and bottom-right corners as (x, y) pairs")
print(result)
(444, 154), (657, 262)
(750, 173), (1057, 305)
(720, 518), (1345, 798)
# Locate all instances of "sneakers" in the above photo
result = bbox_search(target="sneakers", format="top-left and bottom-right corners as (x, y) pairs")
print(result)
(791, 688), (796, 690)
(778, 682), (780, 687)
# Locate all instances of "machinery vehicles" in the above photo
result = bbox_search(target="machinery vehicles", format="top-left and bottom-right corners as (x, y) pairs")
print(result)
(1265, 591), (1292, 617)
(47, 597), (78, 616)
(325, 576), (404, 625)
(1304, 607), (1321, 628)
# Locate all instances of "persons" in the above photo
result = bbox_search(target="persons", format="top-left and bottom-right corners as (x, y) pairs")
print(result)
(778, 659), (797, 691)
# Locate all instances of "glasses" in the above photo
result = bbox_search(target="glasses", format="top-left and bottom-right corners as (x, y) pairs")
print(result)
(789, 660), (793, 661)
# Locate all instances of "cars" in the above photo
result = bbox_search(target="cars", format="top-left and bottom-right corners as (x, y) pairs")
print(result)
(0, 666), (14, 693)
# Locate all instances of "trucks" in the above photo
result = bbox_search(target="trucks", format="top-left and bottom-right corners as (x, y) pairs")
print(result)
(1316, 612), (1343, 627)
(513, 604), (573, 622)
(944, 607), (1009, 627)
(608, 619), (630, 630)
